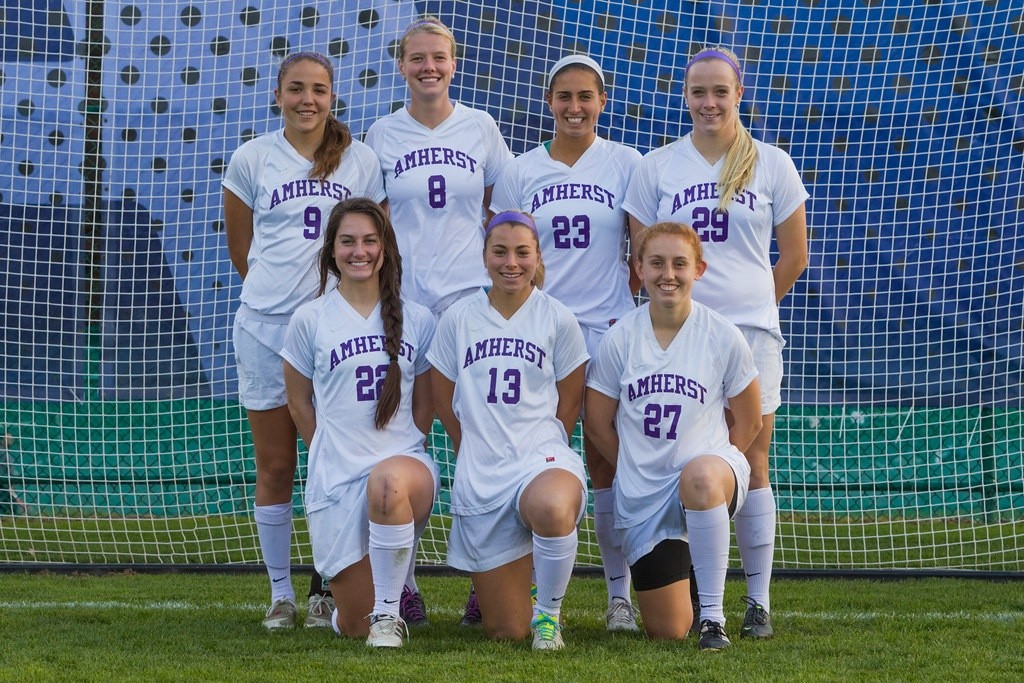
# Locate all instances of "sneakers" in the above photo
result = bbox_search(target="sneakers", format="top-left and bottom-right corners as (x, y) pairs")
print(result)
(303, 595), (337, 628)
(697, 619), (733, 651)
(529, 611), (566, 651)
(262, 600), (296, 631)
(605, 600), (640, 631)
(399, 591), (428, 626)
(460, 585), (482, 625)
(741, 604), (774, 639)
(365, 613), (404, 648)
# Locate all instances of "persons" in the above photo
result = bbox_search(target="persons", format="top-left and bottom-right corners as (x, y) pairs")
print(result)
(624, 46), (813, 639)
(358, 19), (512, 635)
(424, 212), (592, 657)
(287, 196), (437, 652)
(487, 52), (649, 631)
(586, 224), (763, 656)
(217, 50), (388, 635)
